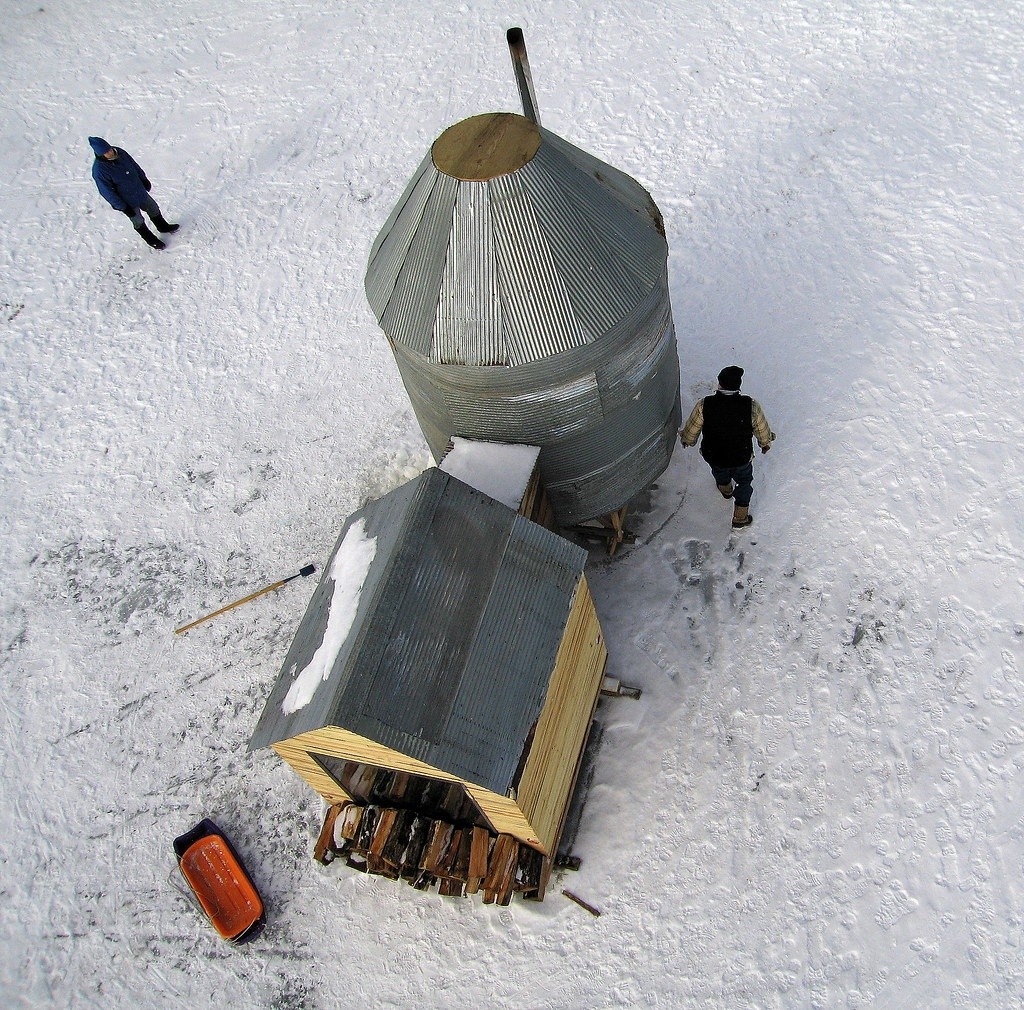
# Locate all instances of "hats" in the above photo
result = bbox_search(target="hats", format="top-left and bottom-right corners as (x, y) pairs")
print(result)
(718, 366), (744, 391)
(89, 137), (111, 157)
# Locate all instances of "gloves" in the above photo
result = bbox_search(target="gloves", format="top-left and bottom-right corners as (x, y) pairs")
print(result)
(142, 178), (151, 192)
(122, 205), (136, 218)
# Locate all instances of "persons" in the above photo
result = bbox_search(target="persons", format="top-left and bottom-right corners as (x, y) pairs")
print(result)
(679, 366), (776, 531)
(88, 137), (179, 251)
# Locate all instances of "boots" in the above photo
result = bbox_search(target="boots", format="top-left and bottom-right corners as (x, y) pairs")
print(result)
(718, 482), (734, 499)
(136, 222), (166, 251)
(151, 213), (179, 233)
(732, 505), (752, 528)
